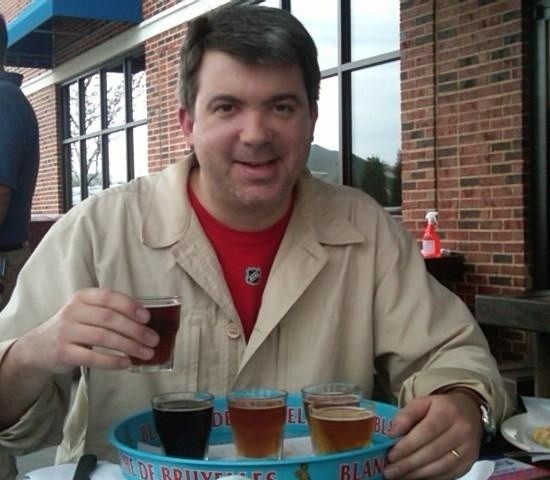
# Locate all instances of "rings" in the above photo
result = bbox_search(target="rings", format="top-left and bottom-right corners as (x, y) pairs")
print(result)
(451, 450), (461, 461)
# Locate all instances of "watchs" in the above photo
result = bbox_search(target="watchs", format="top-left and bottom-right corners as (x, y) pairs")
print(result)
(447, 387), (496, 444)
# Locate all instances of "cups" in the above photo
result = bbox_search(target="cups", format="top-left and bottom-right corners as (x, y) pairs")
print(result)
(306, 400), (374, 456)
(150, 388), (214, 465)
(300, 382), (363, 435)
(124, 295), (183, 373)
(226, 389), (288, 464)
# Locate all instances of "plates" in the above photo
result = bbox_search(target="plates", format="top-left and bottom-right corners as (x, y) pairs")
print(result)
(501, 414), (550, 454)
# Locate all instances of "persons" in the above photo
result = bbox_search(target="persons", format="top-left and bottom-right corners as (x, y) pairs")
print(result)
(0, 13), (40, 312)
(0, 3), (505, 480)
